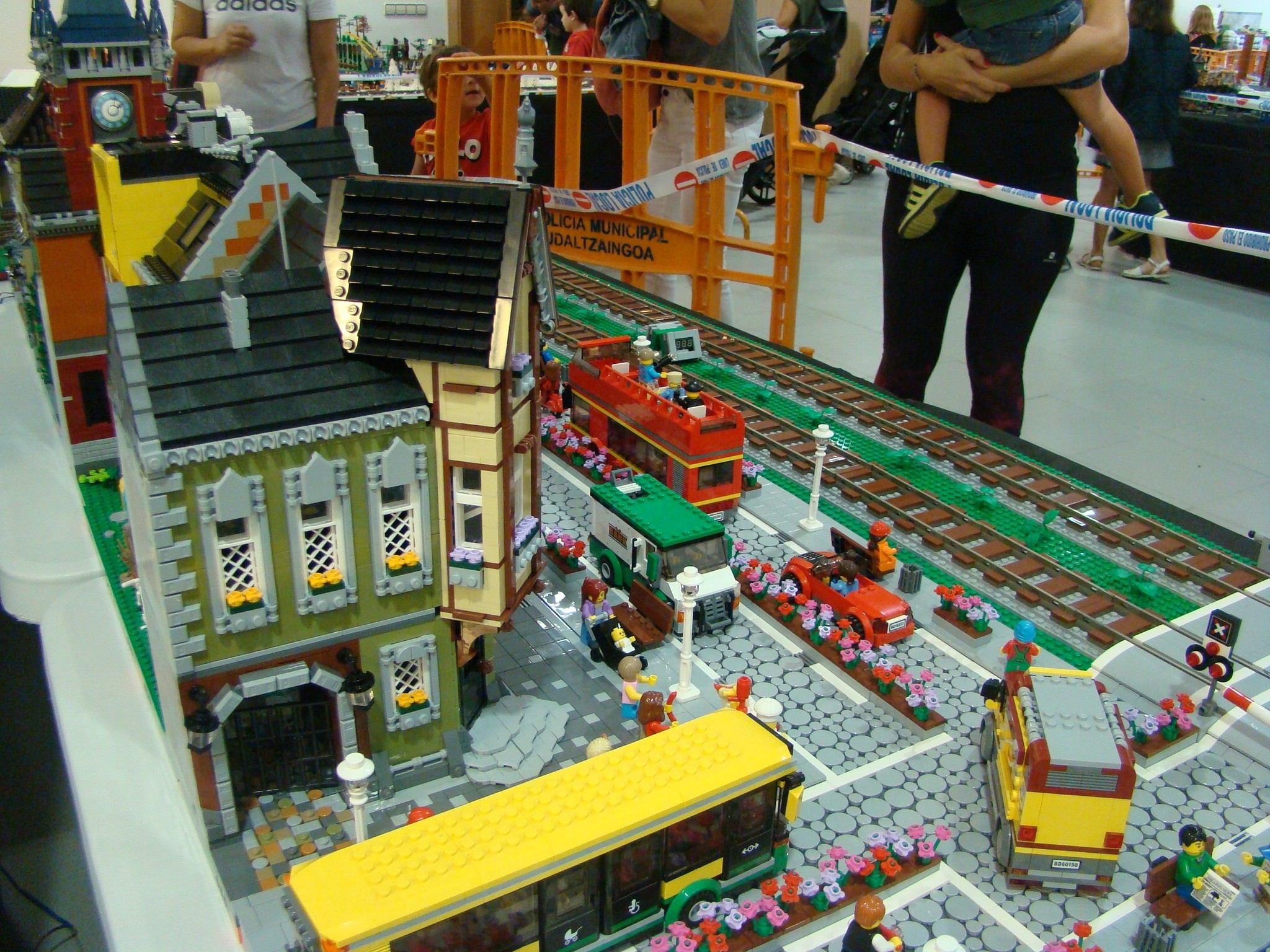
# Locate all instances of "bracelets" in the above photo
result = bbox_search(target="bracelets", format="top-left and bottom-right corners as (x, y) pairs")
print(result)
(913, 53), (927, 87)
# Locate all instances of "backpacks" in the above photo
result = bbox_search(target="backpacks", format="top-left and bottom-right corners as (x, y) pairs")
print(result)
(794, 0), (848, 55)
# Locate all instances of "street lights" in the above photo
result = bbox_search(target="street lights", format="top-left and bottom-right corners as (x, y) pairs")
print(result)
(336, 752), (376, 843)
(668, 566), (706, 702)
(797, 424), (835, 531)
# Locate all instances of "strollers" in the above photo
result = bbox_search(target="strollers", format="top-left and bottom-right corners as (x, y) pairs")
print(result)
(585, 612), (648, 671)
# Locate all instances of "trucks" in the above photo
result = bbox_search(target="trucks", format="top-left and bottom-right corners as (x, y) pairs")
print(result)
(588, 469), (740, 637)
(979, 668), (1136, 893)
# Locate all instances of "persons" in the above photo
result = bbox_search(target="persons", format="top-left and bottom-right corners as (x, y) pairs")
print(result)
(410, 46), (493, 178)
(520, 0), (601, 76)
(841, 895), (902, 952)
(873, 0), (1197, 439)
(171, 0), (338, 132)
(1002, 621), (1041, 675)
(1176, 824), (1229, 913)
(1187, 5), (1216, 50)
(1241, 844), (1270, 914)
(823, 558), (857, 596)
(869, 522), (898, 570)
(540, 340), (561, 415)
(581, 576), (677, 740)
(633, 335), (705, 411)
(768, 0), (871, 167)
(600, 0), (767, 328)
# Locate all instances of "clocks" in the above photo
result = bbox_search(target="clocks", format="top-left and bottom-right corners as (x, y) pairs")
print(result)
(90, 89), (134, 133)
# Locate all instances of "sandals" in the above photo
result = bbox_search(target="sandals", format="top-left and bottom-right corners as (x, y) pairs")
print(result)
(1121, 257), (1172, 278)
(1076, 249), (1104, 271)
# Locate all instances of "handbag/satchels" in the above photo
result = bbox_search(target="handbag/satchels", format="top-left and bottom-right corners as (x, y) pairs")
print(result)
(598, 0), (659, 93)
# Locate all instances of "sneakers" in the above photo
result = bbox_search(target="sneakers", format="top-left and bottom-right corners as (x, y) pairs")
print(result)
(1108, 191), (1169, 247)
(896, 162), (958, 240)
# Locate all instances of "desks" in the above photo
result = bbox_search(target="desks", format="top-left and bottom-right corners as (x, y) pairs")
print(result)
(1120, 111), (1270, 295)
(334, 93), (657, 191)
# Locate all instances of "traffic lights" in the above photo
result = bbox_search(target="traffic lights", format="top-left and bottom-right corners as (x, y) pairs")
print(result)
(1185, 645), (1233, 684)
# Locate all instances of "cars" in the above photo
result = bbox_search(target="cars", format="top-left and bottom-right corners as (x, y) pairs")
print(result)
(780, 551), (915, 647)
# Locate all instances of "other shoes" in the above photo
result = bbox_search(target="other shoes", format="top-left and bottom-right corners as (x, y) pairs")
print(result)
(1059, 257), (1071, 273)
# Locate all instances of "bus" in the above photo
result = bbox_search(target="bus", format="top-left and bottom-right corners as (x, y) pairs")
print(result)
(283, 705), (805, 952)
(562, 337), (746, 523)
